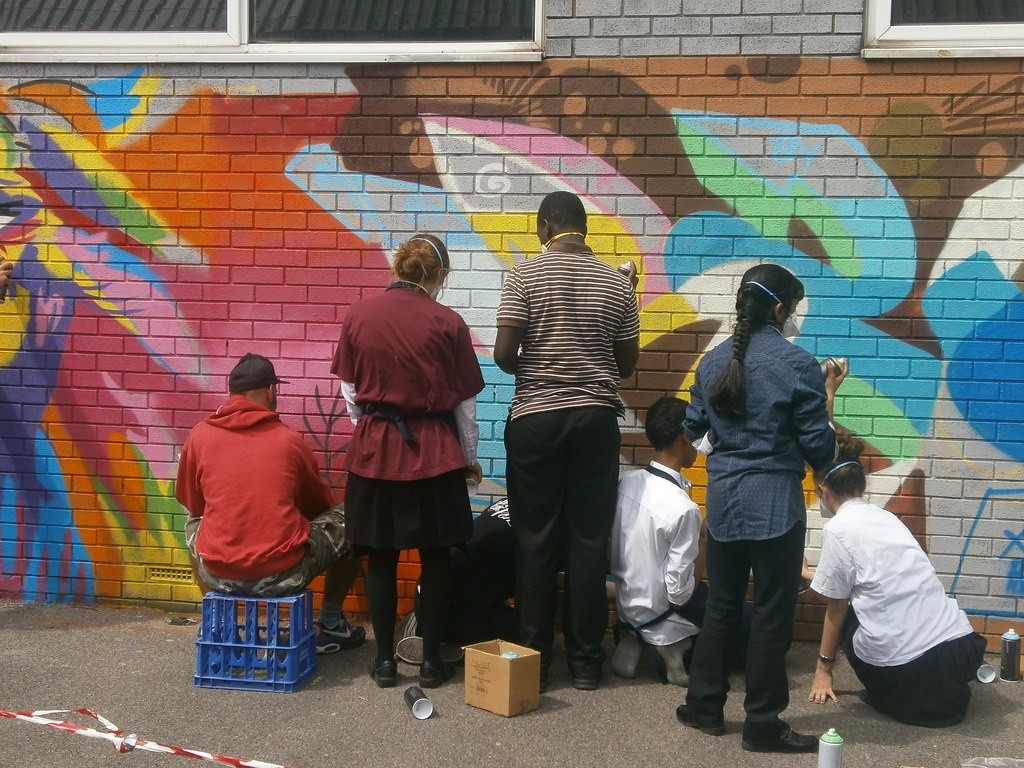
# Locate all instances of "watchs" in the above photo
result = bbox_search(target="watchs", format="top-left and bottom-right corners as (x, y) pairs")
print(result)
(818, 654), (834, 664)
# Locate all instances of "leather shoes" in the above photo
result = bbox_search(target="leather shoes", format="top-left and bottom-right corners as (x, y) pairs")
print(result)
(742, 725), (818, 752)
(677, 705), (726, 735)
(420, 660), (455, 688)
(369, 658), (397, 687)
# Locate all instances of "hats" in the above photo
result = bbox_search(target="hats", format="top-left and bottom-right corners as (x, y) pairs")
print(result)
(229, 353), (291, 394)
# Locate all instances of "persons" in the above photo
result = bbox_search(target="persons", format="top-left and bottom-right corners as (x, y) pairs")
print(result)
(325, 232), (486, 688)
(410, 489), (617, 664)
(174, 351), (366, 655)
(800, 430), (987, 728)
(608, 394), (742, 677)
(676, 264), (850, 753)
(489, 189), (641, 691)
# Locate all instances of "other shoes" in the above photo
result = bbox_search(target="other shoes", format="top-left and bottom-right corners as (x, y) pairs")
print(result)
(393, 609), (417, 660)
(396, 637), (464, 664)
(570, 664), (603, 690)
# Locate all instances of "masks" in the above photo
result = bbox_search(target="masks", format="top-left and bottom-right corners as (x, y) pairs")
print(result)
(819, 499), (835, 518)
(541, 244), (548, 254)
(430, 286), (440, 300)
(782, 311), (800, 337)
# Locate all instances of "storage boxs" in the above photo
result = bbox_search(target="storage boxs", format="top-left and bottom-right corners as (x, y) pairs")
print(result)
(193, 588), (318, 694)
(461, 638), (541, 716)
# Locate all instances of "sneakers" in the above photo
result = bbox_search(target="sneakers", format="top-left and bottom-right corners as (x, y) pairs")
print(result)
(315, 612), (366, 654)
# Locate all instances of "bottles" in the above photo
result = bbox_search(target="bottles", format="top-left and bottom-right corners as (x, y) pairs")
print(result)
(1000, 629), (1022, 683)
(404, 685), (434, 720)
(976, 658), (996, 684)
(818, 728), (844, 768)
(817, 356), (846, 380)
